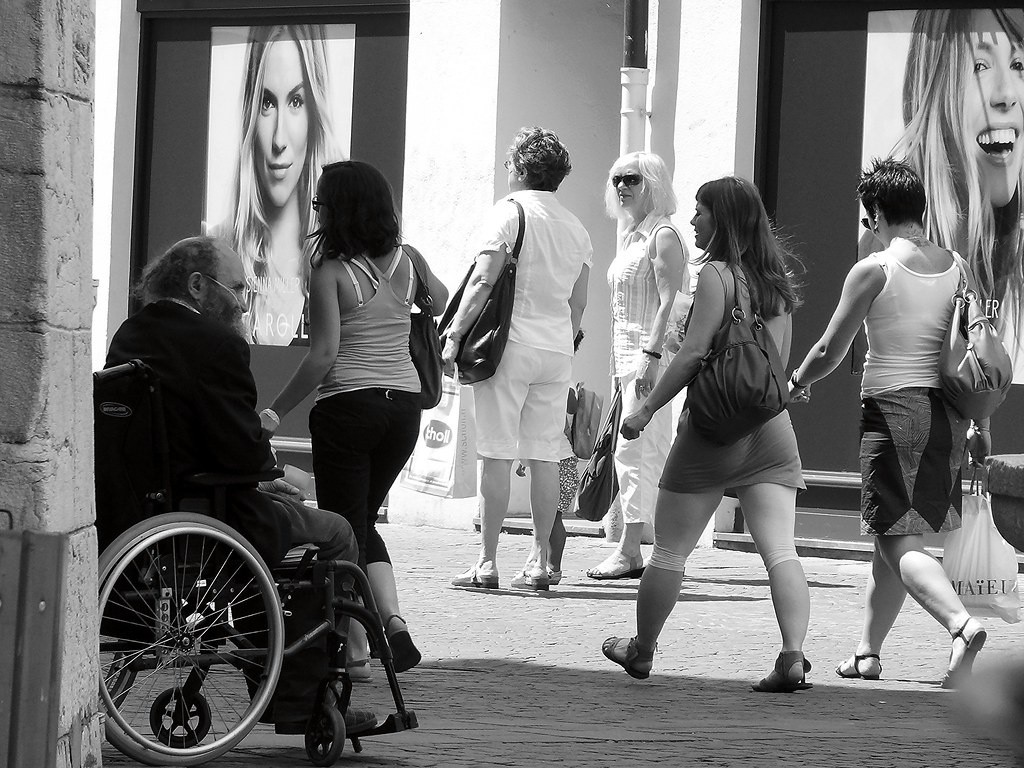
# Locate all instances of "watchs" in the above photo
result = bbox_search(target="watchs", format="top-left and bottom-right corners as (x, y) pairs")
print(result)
(790, 368), (807, 390)
(446, 329), (461, 343)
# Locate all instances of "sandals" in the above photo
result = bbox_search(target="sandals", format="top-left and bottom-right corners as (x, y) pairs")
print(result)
(344, 656), (378, 683)
(750, 649), (816, 693)
(384, 614), (422, 674)
(601, 636), (658, 679)
(512, 568), (552, 589)
(938, 617), (988, 690)
(450, 564), (503, 590)
(545, 568), (566, 582)
(835, 652), (881, 680)
(586, 549), (648, 582)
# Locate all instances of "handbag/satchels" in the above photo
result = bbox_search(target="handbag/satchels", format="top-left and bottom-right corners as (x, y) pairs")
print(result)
(940, 460), (1022, 625)
(674, 258), (793, 445)
(397, 243), (444, 410)
(938, 247), (1013, 421)
(573, 383), (629, 522)
(435, 198), (527, 388)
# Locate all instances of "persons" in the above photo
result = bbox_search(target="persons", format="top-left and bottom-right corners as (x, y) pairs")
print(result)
(516, 329), (586, 584)
(258, 160), (449, 681)
(586, 150), (691, 580)
(441, 127), (595, 590)
(102, 236), (378, 735)
(787, 159), (988, 689)
(858, 10), (1024, 380)
(601, 176), (812, 692)
(206, 24), (346, 347)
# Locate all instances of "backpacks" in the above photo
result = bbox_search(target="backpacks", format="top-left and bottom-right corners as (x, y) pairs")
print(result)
(565, 379), (605, 461)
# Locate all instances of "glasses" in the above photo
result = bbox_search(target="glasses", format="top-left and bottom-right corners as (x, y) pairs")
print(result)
(612, 174), (642, 188)
(503, 160), (518, 169)
(310, 192), (326, 214)
(860, 211), (871, 230)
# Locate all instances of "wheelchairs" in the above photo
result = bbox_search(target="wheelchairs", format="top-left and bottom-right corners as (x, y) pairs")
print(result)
(88, 357), (418, 768)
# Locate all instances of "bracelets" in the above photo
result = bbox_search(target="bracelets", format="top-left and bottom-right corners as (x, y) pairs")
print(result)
(642, 348), (662, 359)
(260, 408), (281, 426)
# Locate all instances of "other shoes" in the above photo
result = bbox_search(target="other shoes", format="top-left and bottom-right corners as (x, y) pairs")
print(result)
(275, 697), (378, 735)
